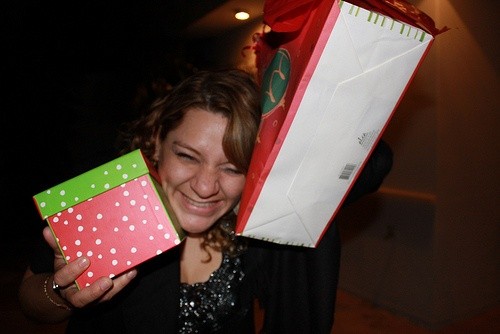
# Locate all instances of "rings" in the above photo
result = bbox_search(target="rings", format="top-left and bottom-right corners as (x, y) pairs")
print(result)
(51, 280), (61, 294)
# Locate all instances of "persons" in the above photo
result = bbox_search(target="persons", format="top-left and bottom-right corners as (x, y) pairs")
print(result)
(4, 66), (338, 334)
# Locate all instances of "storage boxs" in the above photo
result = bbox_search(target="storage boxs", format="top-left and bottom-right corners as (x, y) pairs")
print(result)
(33, 149), (185, 292)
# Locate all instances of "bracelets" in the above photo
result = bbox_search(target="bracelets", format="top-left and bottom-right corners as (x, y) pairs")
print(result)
(43, 273), (74, 314)
